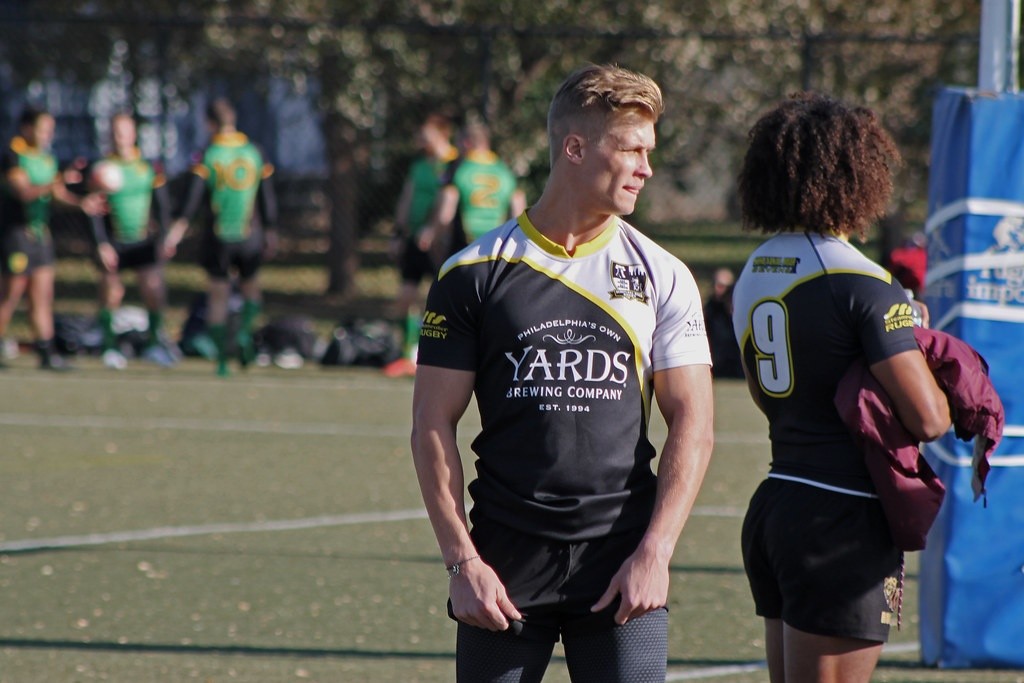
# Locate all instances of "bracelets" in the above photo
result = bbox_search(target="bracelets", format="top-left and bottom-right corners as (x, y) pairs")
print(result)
(445, 555), (479, 575)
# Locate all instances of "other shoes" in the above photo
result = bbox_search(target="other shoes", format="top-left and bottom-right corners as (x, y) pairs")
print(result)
(385, 358), (416, 377)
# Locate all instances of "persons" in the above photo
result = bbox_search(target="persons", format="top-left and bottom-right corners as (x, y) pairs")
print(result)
(729, 92), (953, 683)
(0, 108), (106, 370)
(384, 94), (528, 375)
(155, 98), (279, 375)
(410, 62), (715, 683)
(82, 114), (180, 366)
(703, 265), (746, 379)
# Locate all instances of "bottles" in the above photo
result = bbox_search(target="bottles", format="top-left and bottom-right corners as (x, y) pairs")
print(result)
(904, 289), (922, 328)
